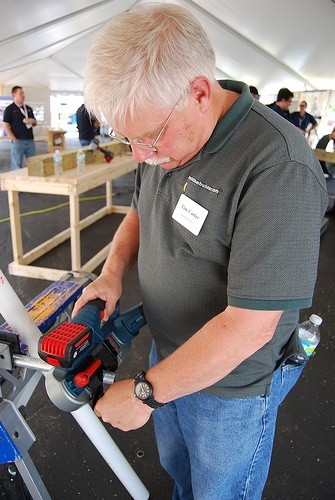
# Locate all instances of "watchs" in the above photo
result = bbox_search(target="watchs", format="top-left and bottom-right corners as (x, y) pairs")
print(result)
(133, 370), (167, 409)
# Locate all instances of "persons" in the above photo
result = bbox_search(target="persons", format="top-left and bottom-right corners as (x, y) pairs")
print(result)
(248, 85), (318, 140)
(2, 86), (38, 171)
(76, 105), (101, 146)
(71, 3), (328, 500)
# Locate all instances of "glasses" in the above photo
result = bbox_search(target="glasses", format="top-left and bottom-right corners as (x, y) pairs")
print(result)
(108, 95), (182, 153)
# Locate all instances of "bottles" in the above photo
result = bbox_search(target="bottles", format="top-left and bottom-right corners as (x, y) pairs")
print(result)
(54, 150), (62, 177)
(288, 314), (323, 364)
(76, 149), (85, 173)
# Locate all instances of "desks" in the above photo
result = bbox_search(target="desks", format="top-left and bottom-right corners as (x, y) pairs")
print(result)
(0, 152), (140, 285)
